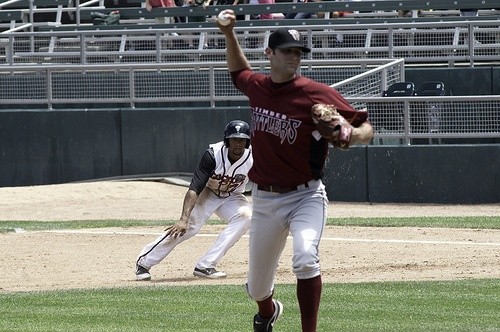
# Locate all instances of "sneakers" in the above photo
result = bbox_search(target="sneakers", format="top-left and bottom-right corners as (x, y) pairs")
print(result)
(193, 266), (227, 278)
(136, 264), (151, 280)
(253, 297), (283, 332)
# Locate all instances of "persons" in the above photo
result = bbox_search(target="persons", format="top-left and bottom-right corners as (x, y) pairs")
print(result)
(217, 10), (373, 332)
(136, 121), (253, 281)
(145, 0), (353, 47)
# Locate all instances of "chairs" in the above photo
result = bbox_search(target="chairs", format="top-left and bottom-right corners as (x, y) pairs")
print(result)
(0, 0), (500, 62)
(366, 81), (446, 146)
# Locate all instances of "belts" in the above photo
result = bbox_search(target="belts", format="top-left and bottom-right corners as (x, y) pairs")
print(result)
(258, 182), (310, 194)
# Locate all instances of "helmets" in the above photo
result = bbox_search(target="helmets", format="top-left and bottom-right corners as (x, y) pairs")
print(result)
(224, 119), (251, 148)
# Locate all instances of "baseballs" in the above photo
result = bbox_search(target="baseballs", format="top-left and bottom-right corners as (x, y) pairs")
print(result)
(218, 10), (230, 26)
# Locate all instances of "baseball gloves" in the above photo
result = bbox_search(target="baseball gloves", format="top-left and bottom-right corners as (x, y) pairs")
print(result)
(311, 103), (353, 152)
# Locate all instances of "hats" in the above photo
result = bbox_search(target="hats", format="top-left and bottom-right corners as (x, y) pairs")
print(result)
(268, 29), (311, 53)
